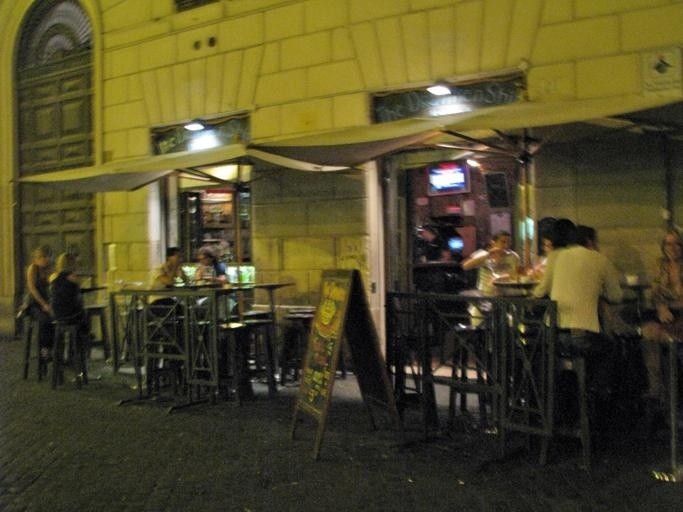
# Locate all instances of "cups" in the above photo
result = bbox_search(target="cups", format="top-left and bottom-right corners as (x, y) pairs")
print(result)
(627, 275), (639, 286)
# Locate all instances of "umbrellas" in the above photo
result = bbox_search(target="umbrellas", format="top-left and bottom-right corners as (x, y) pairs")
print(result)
(246, 91), (683, 266)
(8, 132), (352, 323)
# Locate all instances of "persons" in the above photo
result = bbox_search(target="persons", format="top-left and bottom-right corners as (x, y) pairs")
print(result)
(194, 246), (228, 284)
(147, 247), (189, 317)
(419, 218), (625, 334)
(641, 225), (683, 401)
(23, 244), (95, 385)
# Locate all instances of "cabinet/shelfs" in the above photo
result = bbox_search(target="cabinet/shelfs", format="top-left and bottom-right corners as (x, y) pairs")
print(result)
(201, 198), (250, 261)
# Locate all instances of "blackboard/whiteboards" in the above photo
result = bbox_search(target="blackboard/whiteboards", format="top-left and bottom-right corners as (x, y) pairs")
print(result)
(297, 269), (352, 422)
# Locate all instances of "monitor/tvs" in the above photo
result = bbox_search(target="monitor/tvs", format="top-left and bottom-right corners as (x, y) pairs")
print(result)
(425, 166), (471, 196)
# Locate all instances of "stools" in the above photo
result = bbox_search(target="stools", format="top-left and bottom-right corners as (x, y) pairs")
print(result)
(22, 306), (110, 390)
(401, 328), (595, 469)
(166, 308), (318, 403)
